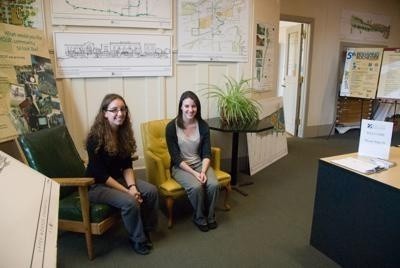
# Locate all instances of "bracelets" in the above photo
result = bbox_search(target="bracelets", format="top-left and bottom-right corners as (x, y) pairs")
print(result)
(126, 184), (136, 189)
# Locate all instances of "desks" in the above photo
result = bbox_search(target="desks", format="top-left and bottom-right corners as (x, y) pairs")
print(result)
(309, 144), (399, 267)
(205, 117), (274, 197)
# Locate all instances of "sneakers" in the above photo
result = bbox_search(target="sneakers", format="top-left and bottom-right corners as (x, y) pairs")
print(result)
(198, 221), (217, 232)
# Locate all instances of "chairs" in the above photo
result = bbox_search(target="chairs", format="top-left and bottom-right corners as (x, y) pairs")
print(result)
(15, 124), (120, 261)
(141, 119), (233, 228)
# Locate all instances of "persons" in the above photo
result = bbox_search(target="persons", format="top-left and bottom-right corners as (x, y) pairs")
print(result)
(165, 90), (220, 231)
(78, 93), (161, 255)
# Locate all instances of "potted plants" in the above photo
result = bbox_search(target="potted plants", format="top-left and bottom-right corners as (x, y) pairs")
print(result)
(194, 71), (266, 135)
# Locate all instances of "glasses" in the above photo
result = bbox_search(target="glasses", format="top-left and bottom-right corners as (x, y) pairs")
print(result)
(106, 108), (128, 114)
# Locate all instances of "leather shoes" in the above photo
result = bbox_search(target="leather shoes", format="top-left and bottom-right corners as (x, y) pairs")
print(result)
(130, 230), (153, 254)
